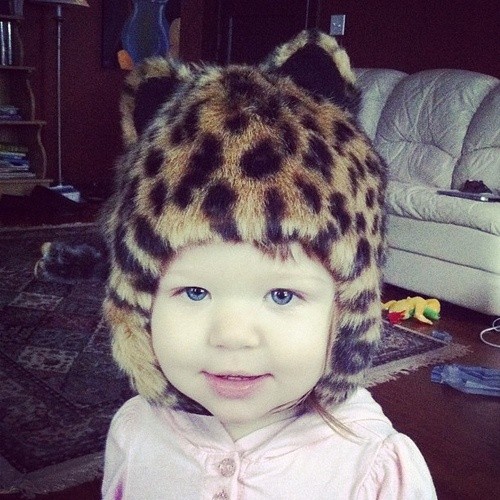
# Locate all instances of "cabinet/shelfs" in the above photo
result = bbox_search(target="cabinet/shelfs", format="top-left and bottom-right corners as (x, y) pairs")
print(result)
(0, 0), (53, 208)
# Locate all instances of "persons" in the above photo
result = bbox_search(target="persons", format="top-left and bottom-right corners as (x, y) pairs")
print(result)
(36, 30), (437, 500)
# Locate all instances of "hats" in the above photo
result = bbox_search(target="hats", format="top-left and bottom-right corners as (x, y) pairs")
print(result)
(36, 30), (387, 408)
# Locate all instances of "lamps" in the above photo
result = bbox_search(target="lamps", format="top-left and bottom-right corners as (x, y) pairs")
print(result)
(43, 0), (89, 188)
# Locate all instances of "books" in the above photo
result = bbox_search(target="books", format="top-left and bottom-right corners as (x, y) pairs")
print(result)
(0, 144), (35, 179)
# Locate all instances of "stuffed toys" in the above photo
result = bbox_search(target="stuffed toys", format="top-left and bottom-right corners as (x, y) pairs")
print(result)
(381, 296), (440, 325)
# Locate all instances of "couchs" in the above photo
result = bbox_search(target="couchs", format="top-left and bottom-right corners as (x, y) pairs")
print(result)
(332, 68), (500, 318)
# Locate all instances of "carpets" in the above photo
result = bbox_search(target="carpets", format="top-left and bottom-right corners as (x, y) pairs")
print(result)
(0, 223), (470, 500)
